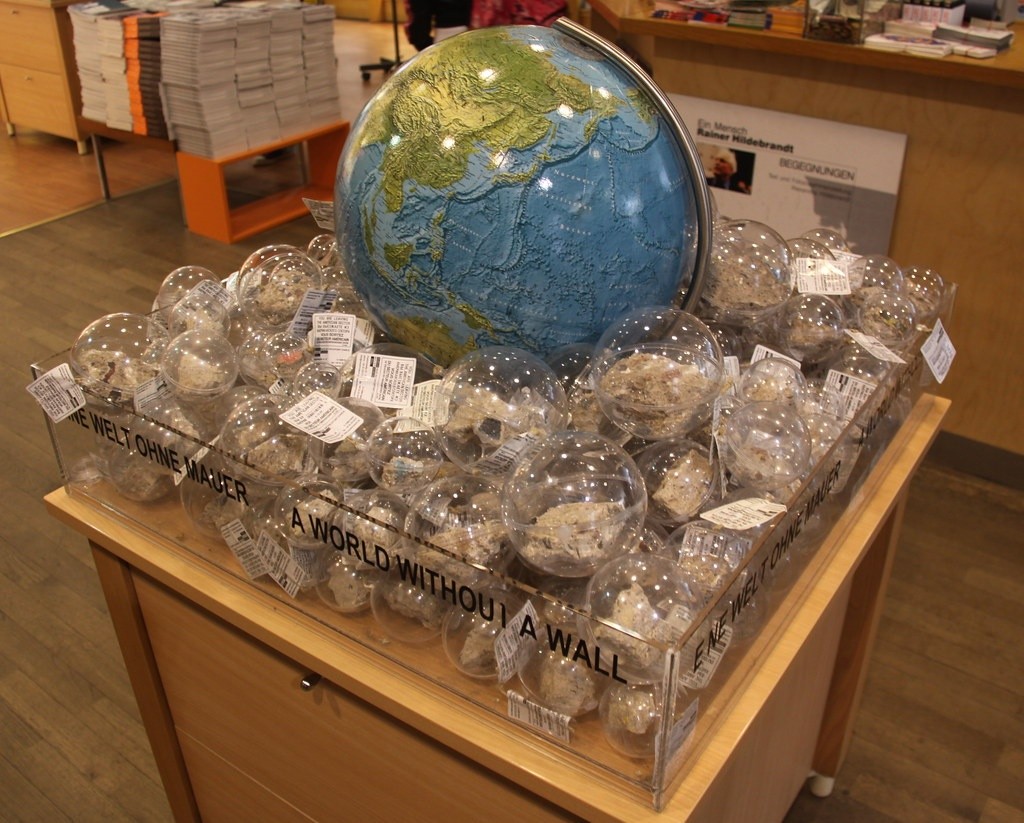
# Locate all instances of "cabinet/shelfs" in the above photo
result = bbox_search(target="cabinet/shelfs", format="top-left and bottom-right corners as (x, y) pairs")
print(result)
(42, 393), (954, 823)
(178, 117), (351, 245)
(0, 0), (88, 153)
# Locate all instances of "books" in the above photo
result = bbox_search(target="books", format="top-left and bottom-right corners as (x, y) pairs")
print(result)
(66, 0), (343, 161)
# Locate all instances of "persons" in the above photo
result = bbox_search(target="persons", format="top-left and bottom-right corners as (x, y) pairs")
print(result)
(706, 149), (745, 193)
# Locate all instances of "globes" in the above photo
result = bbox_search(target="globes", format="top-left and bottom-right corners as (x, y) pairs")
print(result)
(333, 13), (712, 395)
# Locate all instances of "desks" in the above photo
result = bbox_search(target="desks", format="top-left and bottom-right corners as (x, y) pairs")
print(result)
(83, 113), (185, 228)
